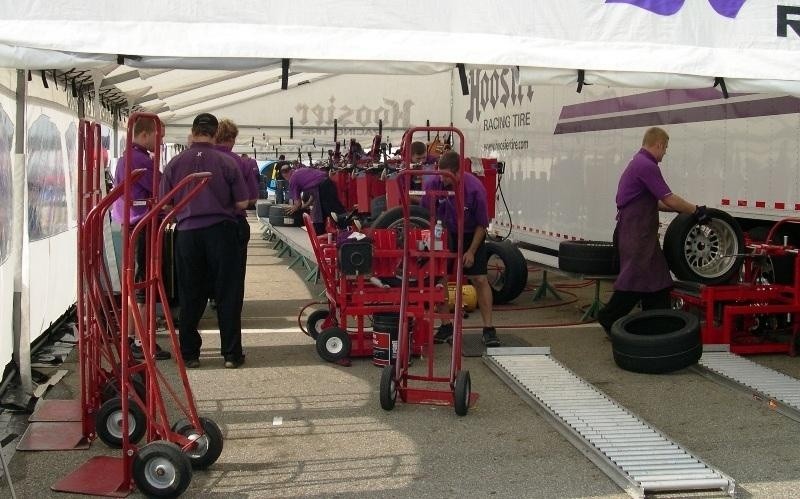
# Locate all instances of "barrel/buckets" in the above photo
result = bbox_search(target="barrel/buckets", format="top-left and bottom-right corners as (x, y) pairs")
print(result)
(372, 313), (415, 368)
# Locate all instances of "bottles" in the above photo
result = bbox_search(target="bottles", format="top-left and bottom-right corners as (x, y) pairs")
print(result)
(434, 220), (443, 241)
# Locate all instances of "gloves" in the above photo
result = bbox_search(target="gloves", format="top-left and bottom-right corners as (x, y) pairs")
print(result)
(691, 205), (713, 226)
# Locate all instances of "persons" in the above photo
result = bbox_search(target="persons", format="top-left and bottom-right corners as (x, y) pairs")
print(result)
(109, 111), (173, 359)
(274, 135), (432, 249)
(202, 117), (251, 315)
(597, 127), (712, 341)
(420, 151), (502, 350)
(156, 111), (251, 371)
(240, 154), (260, 210)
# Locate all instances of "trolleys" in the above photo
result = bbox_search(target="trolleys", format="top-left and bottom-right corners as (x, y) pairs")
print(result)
(376, 124), (474, 418)
(292, 206), (452, 364)
(17, 119), (148, 451)
(42, 112), (224, 497)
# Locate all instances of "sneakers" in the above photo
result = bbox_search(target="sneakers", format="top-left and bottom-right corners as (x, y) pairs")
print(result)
(432, 323), (454, 342)
(127, 336), (245, 368)
(480, 326), (500, 346)
(598, 309), (614, 336)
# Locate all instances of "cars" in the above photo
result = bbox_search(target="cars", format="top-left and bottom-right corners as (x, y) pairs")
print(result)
(256, 159), (307, 196)
